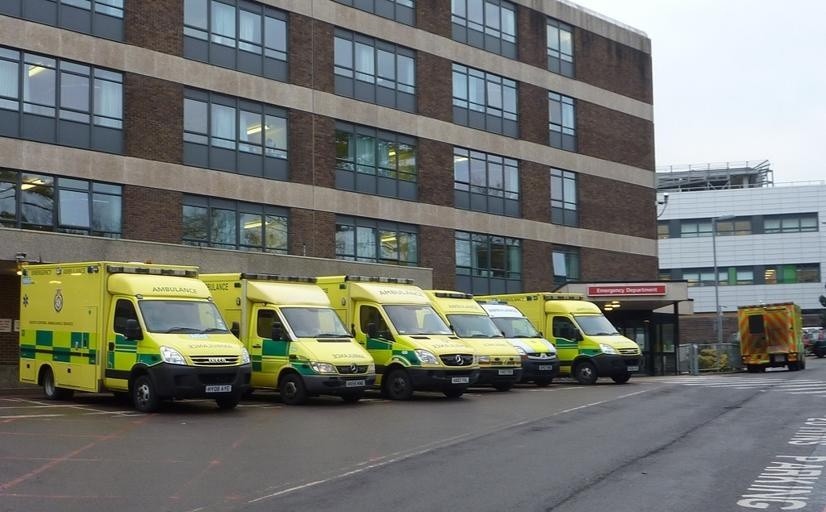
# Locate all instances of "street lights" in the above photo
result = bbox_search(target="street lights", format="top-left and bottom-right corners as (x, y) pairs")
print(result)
(712, 213), (736, 342)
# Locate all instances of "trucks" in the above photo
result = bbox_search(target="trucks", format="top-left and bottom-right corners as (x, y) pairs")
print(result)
(422, 289), (643, 392)
(315, 273), (482, 400)
(14, 249), (254, 416)
(198, 270), (377, 406)
(736, 301), (807, 371)
